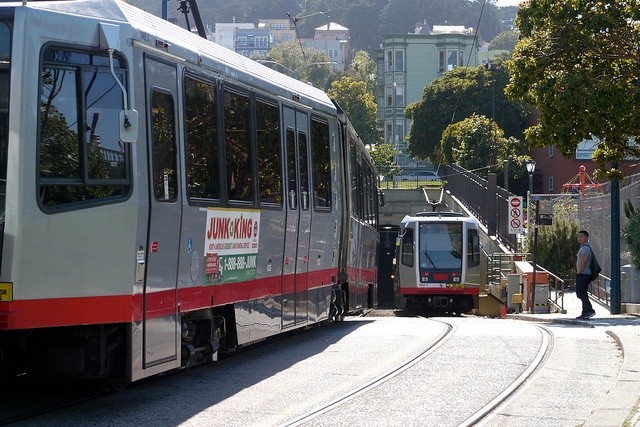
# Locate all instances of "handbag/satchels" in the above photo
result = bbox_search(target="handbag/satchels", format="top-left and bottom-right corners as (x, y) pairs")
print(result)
(583, 244), (601, 281)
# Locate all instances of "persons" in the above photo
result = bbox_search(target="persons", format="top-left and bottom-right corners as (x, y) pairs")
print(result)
(575, 230), (596, 319)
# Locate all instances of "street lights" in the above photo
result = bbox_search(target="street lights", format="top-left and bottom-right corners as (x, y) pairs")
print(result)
(525, 159), (536, 193)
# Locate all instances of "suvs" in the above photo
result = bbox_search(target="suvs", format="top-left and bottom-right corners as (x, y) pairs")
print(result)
(393, 170), (442, 181)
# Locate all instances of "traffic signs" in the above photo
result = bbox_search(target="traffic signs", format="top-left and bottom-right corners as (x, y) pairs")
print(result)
(508, 196), (523, 235)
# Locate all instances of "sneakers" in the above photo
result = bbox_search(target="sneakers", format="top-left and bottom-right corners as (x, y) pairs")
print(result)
(582, 310), (596, 319)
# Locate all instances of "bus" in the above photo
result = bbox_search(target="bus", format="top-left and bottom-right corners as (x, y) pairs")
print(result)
(0, 0), (384, 421)
(390, 211), (482, 317)
